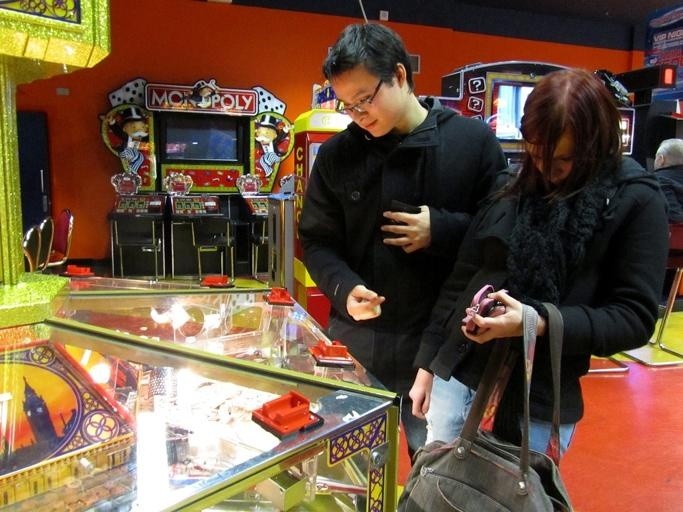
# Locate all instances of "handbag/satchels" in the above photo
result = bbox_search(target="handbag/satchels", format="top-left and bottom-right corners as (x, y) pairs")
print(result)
(397, 298), (569, 512)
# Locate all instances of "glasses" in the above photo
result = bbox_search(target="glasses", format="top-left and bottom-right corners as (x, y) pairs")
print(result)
(335, 79), (383, 113)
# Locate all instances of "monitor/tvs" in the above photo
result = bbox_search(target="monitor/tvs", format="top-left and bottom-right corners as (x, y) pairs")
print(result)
(618, 108), (633, 154)
(308, 140), (325, 177)
(492, 81), (536, 141)
(155, 112), (243, 164)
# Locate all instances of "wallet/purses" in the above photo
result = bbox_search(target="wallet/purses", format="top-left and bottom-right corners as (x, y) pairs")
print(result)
(462, 284), (510, 336)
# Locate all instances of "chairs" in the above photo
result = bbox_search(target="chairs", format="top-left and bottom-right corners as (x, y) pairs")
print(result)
(21, 207), (74, 278)
(249, 211), (275, 280)
(619, 221), (682, 369)
(190, 214), (236, 283)
(113, 209), (163, 280)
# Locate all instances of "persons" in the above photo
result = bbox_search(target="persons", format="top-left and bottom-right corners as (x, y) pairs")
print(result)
(410, 68), (670, 461)
(298, 24), (509, 447)
(653, 135), (682, 300)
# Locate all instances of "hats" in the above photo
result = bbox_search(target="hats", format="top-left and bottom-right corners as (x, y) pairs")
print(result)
(196, 81), (206, 89)
(259, 114), (278, 130)
(123, 107), (143, 121)
